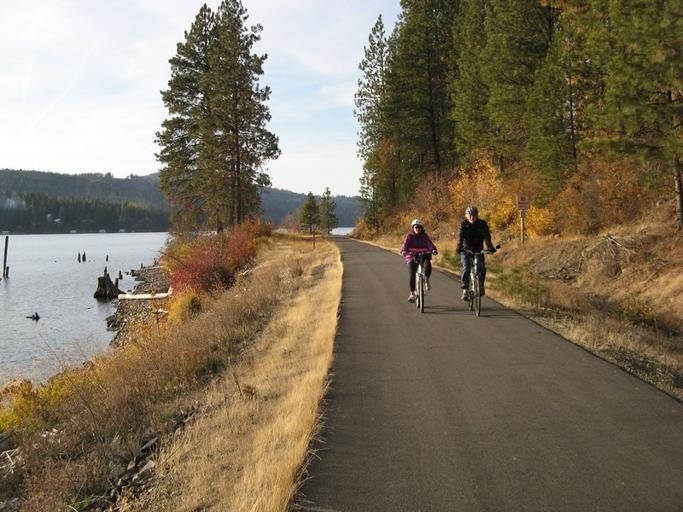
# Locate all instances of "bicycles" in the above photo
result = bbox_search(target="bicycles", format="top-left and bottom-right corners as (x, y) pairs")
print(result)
(401, 251), (437, 313)
(455, 245), (500, 318)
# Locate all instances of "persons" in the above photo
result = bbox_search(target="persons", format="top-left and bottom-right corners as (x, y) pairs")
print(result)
(456, 206), (496, 302)
(399, 219), (438, 303)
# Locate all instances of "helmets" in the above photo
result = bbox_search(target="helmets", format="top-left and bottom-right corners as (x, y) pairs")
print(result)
(464, 206), (479, 214)
(410, 219), (423, 228)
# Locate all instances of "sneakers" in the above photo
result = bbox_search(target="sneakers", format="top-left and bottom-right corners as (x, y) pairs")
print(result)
(479, 286), (486, 296)
(460, 289), (471, 301)
(408, 294), (417, 303)
(424, 279), (431, 292)
